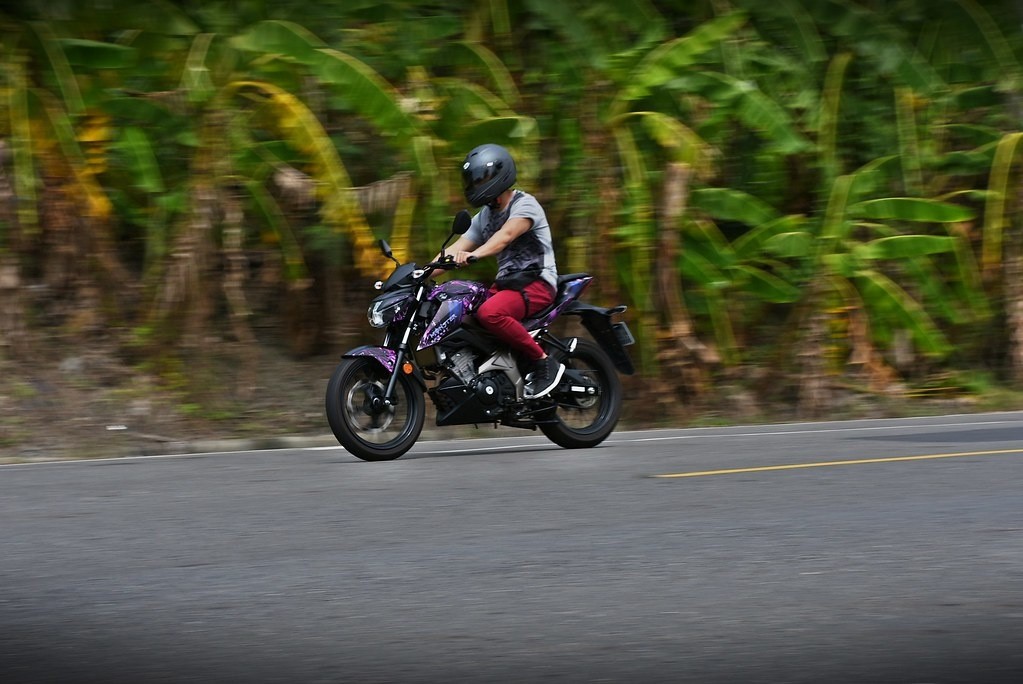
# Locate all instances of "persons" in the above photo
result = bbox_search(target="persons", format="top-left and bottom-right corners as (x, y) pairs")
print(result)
(419, 142), (567, 399)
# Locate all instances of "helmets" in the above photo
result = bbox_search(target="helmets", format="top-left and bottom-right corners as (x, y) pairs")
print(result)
(462, 143), (518, 208)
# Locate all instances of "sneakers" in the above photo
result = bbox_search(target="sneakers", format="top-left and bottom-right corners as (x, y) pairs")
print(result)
(531, 354), (566, 400)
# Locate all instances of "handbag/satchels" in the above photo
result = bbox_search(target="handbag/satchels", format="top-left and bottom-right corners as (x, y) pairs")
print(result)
(495, 261), (542, 292)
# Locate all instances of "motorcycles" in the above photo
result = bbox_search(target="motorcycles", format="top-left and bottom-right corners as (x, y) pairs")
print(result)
(326, 209), (637, 460)
